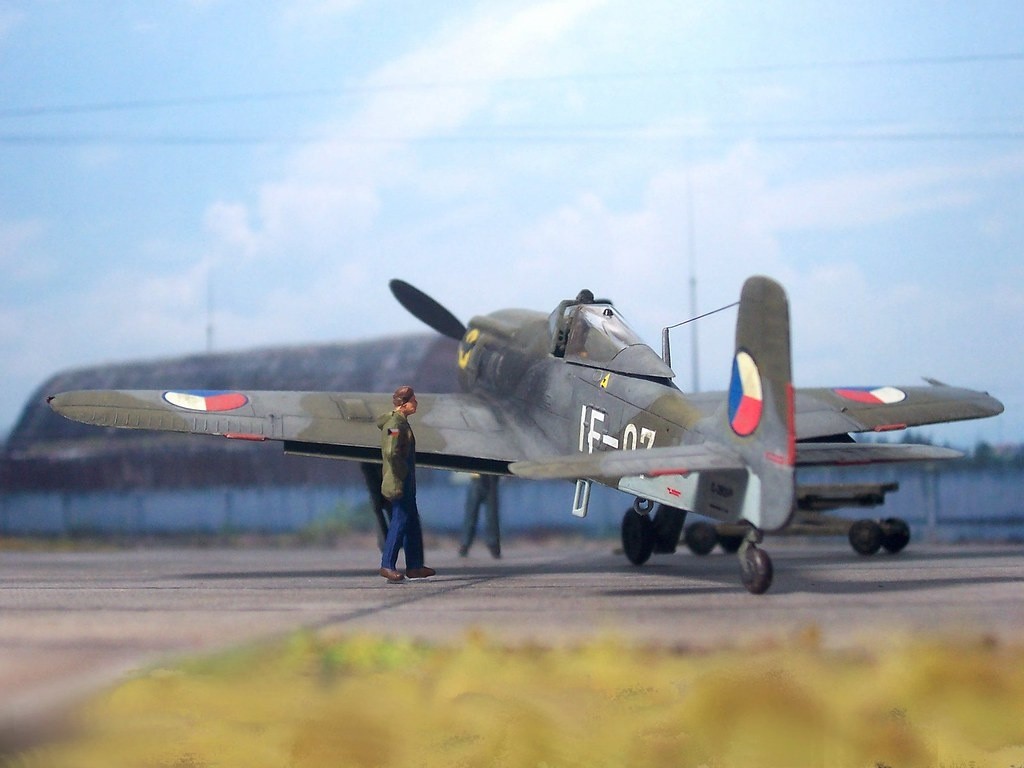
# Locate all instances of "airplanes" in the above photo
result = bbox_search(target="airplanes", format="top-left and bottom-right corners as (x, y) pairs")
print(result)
(46, 275), (1004, 595)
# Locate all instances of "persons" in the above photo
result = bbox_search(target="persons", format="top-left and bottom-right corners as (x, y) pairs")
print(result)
(458, 473), (501, 558)
(376, 385), (435, 581)
